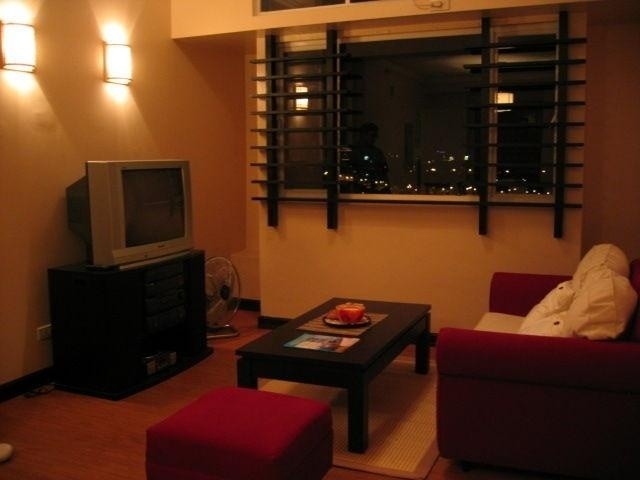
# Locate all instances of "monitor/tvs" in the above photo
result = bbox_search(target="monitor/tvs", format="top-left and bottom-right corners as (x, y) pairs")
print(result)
(66, 160), (193, 268)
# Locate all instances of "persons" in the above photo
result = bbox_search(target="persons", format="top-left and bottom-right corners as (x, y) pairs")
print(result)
(341, 121), (393, 195)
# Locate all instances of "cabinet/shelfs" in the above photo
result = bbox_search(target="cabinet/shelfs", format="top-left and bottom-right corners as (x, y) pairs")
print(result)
(47, 248), (215, 402)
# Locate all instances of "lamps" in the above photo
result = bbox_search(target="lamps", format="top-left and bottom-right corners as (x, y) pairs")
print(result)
(0, 20), (38, 73)
(100, 41), (136, 86)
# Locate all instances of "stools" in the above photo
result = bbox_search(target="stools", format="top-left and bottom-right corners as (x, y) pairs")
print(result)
(143, 384), (335, 479)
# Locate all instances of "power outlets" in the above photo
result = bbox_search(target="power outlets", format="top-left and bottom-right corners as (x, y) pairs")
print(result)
(36, 324), (51, 342)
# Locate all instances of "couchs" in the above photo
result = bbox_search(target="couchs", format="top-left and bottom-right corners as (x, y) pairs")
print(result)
(433, 256), (639, 478)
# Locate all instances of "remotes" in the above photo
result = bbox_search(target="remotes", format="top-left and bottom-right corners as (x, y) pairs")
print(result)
(321, 337), (342, 351)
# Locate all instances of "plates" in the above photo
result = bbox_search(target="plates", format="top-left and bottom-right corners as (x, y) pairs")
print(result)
(322, 314), (372, 329)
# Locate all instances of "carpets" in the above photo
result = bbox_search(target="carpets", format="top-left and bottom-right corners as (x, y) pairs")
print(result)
(257, 354), (441, 479)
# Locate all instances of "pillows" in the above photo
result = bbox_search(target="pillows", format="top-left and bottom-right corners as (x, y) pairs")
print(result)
(518, 242), (639, 340)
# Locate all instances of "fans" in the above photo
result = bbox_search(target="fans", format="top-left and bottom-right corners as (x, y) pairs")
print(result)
(204, 253), (243, 341)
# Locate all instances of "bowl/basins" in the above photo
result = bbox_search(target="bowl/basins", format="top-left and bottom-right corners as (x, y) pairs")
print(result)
(335, 303), (365, 322)
